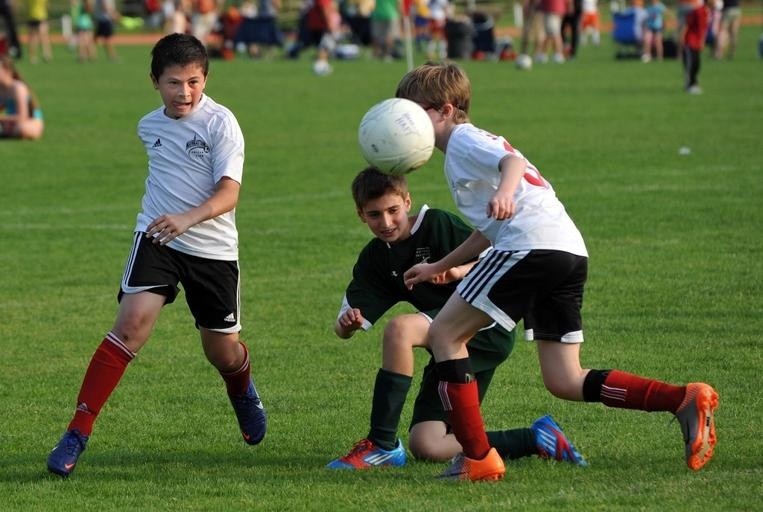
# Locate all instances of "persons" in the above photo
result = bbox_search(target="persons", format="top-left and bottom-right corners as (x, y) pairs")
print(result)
(45, 32), (268, 477)
(323, 166), (587, 471)
(1, 2), (125, 141)
(144, 1), (743, 94)
(393, 58), (720, 484)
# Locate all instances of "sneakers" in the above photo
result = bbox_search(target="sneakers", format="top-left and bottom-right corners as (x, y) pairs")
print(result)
(669, 379), (720, 473)
(430, 452), (506, 487)
(44, 427), (91, 478)
(323, 436), (405, 475)
(522, 412), (590, 470)
(224, 378), (269, 448)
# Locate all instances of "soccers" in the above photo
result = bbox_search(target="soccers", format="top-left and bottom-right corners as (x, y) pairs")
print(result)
(358, 98), (436, 177)
(514, 53), (532, 69)
